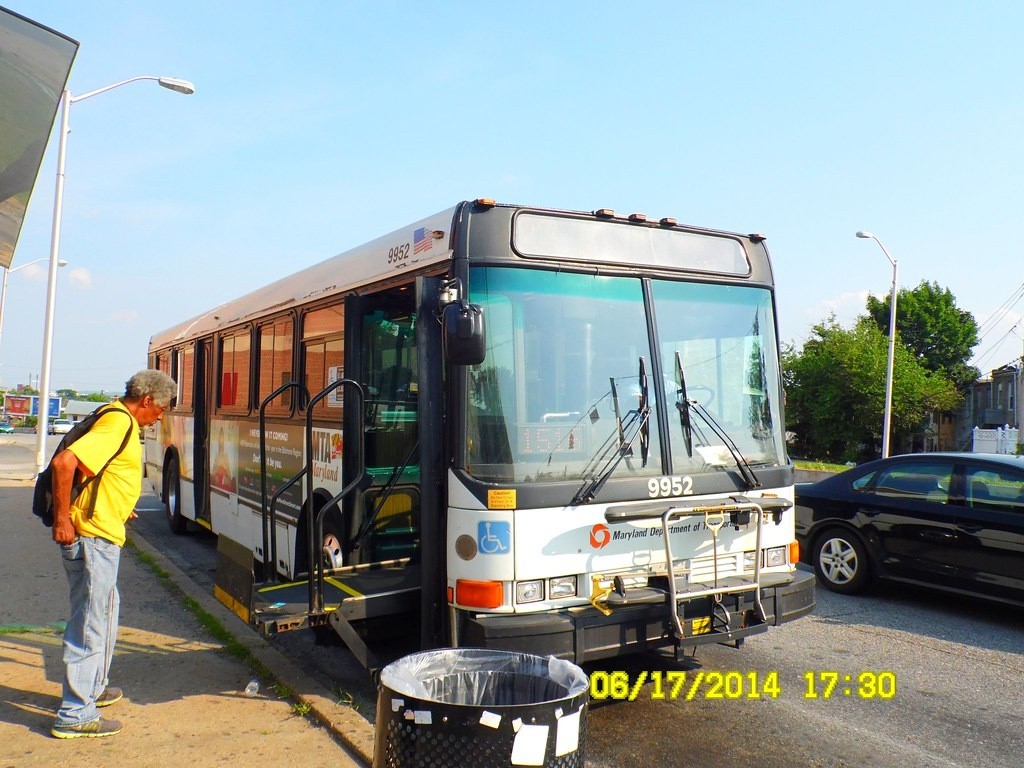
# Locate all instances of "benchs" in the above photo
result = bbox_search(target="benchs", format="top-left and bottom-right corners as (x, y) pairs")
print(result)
(880, 477), (938, 494)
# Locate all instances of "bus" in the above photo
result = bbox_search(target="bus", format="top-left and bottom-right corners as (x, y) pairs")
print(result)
(142, 197), (817, 689)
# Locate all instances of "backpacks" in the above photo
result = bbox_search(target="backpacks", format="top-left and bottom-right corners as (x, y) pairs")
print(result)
(32, 403), (133, 528)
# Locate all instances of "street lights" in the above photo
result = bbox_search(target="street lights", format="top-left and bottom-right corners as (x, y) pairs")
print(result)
(34, 74), (196, 475)
(855, 231), (899, 458)
(0, 257), (70, 336)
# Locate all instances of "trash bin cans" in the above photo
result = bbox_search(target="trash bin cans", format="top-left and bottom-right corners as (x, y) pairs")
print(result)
(370, 647), (593, 768)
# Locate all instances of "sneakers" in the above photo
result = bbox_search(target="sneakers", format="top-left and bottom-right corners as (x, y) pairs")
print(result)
(95, 687), (123, 706)
(49, 717), (122, 739)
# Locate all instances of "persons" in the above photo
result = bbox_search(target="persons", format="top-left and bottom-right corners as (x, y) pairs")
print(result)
(630, 347), (703, 415)
(50, 368), (178, 739)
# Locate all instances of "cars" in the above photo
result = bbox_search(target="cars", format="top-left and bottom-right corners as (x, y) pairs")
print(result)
(794, 451), (1024, 606)
(0, 421), (14, 435)
(72, 421), (81, 425)
(47, 418), (74, 436)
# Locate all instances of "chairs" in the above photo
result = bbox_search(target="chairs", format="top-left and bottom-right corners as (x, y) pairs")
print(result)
(972, 481), (990, 498)
